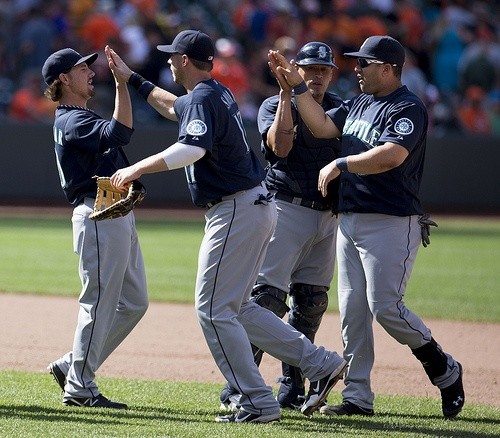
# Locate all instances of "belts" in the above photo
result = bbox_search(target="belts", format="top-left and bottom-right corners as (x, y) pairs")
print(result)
(206, 197), (222, 210)
(275, 191), (329, 212)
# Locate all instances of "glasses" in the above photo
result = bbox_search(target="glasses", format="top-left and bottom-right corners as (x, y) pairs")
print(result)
(356, 57), (397, 68)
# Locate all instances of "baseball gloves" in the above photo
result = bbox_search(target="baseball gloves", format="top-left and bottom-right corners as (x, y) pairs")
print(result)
(88, 176), (146, 221)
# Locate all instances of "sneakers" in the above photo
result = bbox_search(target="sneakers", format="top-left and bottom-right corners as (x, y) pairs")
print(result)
(440, 361), (465, 419)
(214, 409), (281, 424)
(46, 361), (66, 393)
(319, 400), (374, 417)
(301, 360), (349, 416)
(62, 393), (128, 410)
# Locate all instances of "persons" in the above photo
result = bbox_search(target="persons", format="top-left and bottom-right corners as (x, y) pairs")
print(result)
(42, 44), (150, 409)
(269, 35), (465, 419)
(0, 0), (500, 140)
(108, 29), (349, 427)
(220, 41), (345, 413)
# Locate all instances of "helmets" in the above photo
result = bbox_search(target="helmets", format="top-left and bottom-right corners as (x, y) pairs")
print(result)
(295, 42), (339, 71)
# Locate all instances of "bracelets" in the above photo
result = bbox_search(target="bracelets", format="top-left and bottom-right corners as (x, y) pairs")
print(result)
(293, 80), (309, 95)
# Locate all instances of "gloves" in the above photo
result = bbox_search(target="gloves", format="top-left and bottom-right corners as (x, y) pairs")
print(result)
(253, 192), (274, 206)
(418, 213), (438, 248)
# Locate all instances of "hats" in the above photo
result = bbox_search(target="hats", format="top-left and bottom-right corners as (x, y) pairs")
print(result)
(157, 30), (215, 65)
(41, 48), (99, 85)
(343, 35), (406, 68)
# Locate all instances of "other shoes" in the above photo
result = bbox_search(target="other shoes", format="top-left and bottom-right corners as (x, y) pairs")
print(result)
(282, 395), (328, 410)
(219, 394), (242, 410)
